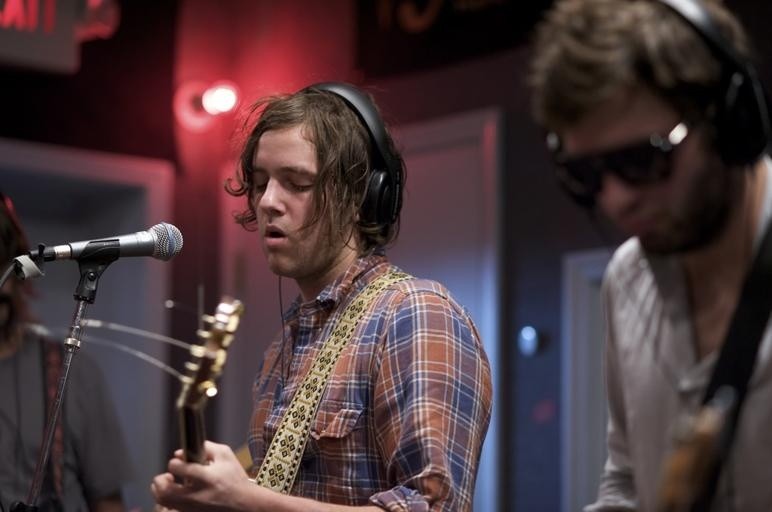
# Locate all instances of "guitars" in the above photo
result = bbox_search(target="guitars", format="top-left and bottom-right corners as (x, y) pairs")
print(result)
(171, 299), (244, 487)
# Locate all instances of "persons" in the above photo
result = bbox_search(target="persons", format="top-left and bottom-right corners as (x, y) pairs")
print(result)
(2, 194), (137, 511)
(151, 80), (493, 511)
(525, 1), (770, 511)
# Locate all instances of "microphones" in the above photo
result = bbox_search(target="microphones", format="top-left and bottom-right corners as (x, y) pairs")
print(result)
(28, 222), (184, 262)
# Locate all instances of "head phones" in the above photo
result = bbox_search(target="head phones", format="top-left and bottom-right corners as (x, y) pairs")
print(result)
(298, 82), (403, 228)
(662, 0), (771, 164)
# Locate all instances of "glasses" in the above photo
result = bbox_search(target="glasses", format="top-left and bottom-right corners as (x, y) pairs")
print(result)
(544, 116), (690, 202)
